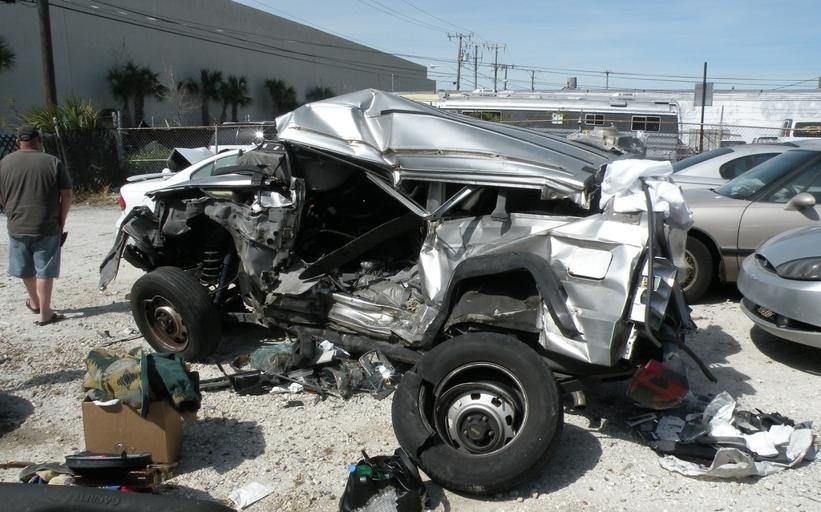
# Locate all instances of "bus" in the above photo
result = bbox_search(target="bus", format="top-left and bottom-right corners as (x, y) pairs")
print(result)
(429, 97), (680, 163)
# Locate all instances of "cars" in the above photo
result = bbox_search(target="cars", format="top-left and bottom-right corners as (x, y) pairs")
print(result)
(669, 119), (821, 349)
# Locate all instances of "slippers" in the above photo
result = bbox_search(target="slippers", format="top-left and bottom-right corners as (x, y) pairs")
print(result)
(26, 299), (64, 325)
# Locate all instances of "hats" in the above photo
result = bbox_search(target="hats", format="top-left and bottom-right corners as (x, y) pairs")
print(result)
(15, 125), (39, 141)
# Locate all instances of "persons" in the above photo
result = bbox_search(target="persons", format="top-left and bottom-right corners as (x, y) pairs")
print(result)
(0, 125), (73, 328)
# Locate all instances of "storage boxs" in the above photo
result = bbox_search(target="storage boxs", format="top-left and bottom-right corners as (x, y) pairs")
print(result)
(82, 362), (197, 463)
